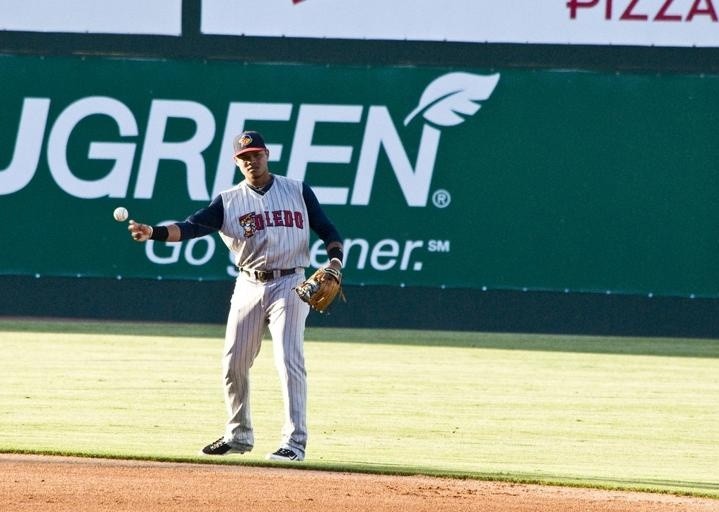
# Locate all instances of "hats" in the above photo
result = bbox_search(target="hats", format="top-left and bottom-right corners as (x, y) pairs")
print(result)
(232, 131), (266, 157)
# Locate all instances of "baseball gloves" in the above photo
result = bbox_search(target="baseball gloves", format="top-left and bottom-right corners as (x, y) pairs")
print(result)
(297, 266), (343, 313)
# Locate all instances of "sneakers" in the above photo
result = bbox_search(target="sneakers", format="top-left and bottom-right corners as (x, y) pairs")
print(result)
(201, 435), (246, 456)
(268, 448), (303, 461)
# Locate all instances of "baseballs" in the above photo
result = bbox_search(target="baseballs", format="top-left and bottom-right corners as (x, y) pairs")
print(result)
(114, 207), (128, 222)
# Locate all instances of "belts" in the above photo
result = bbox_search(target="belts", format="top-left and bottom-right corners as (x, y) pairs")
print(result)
(241, 266), (295, 282)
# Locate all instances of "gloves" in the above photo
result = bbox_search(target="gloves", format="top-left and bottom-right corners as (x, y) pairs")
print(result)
(129, 220), (153, 242)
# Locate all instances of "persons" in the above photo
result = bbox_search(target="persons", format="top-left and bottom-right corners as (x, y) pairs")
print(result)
(127, 129), (345, 463)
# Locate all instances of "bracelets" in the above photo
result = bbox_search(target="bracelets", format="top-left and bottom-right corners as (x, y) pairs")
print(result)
(149, 224), (170, 242)
(327, 247), (344, 265)
